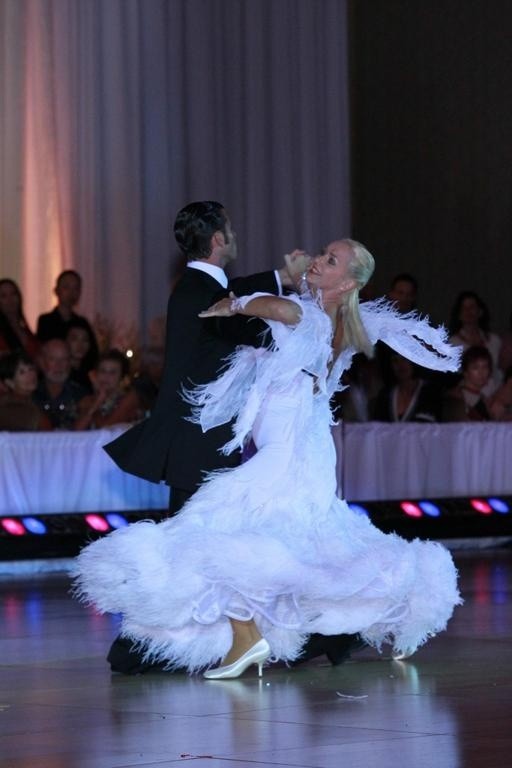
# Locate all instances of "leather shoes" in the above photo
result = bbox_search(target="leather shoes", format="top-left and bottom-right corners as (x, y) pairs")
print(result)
(327, 630), (372, 664)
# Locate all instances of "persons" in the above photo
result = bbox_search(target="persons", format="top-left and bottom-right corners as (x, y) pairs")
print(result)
(337, 277), (512, 423)
(1, 272), (146, 430)
(197, 238), (376, 680)
(102, 198), (371, 676)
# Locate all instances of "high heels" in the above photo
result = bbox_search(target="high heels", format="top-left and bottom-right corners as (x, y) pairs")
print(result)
(391, 621), (436, 661)
(200, 639), (270, 679)
